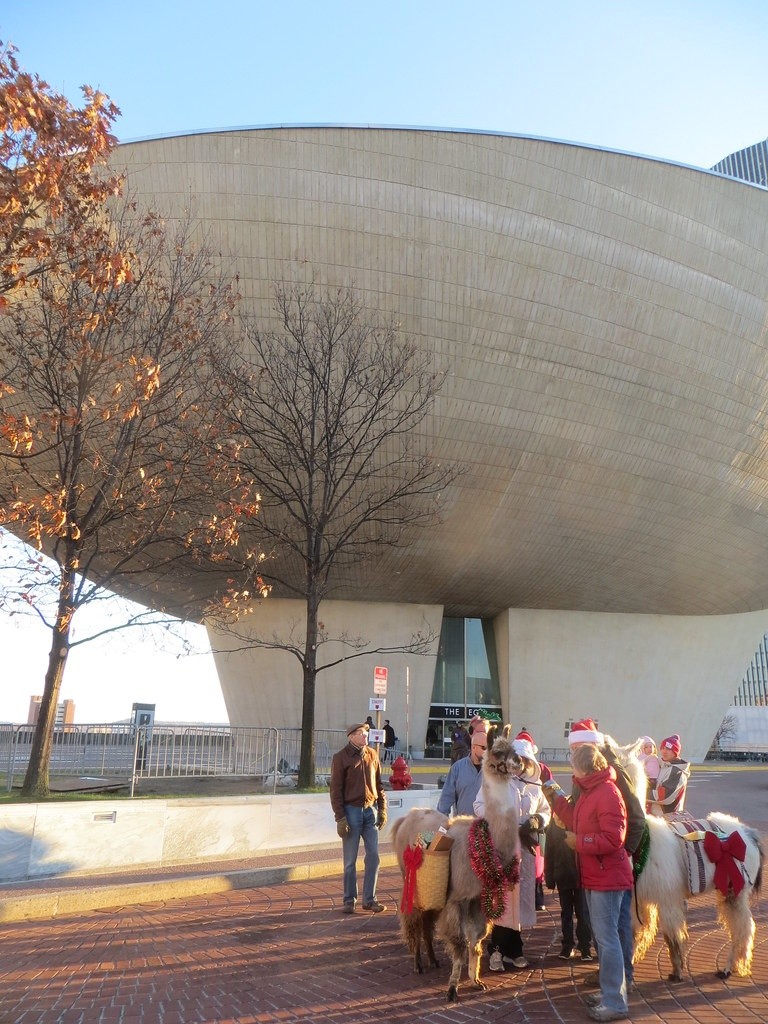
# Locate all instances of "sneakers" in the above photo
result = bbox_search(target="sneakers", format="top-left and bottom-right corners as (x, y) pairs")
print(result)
(503, 956), (529, 968)
(489, 946), (505, 971)
(584, 991), (628, 1021)
(559, 948), (574, 959)
(581, 950), (593, 960)
(344, 901), (356, 912)
(362, 901), (387, 913)
(584, 970), (636, 993)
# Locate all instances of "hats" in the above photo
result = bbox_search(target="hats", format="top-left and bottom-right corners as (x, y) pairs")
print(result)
(513, 733), (538, 754)
(569, 719), (603, 746)
(660, 734), (681, 758)
(346, 723), (369, 737)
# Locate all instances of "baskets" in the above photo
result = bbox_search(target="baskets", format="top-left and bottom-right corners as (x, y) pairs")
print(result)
(407, 842), (450, 912)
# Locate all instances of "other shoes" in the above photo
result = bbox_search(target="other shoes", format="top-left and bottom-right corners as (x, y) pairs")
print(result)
(537, 904), (545, 910)
(684, 899), (689, 912)
(381, 757), (393, 765)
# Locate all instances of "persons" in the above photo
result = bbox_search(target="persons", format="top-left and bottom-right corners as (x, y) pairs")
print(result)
(380, 720), (396, 764)
(330, 723), (387, 914)
(364, 717), (376, 747)
(436, 716), (690, 1022)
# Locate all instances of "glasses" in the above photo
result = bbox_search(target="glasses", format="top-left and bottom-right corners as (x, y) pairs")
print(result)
(367, 719), (372, 720)
(472, 744), (488, 750)
(353, 732), (369, 737)
(384, 722), (388, 724)
(660, 748), (673, 751)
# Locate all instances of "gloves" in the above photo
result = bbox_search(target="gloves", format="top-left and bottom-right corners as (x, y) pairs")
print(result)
(375, 809), (388, 831)
(337, 816), (350, 839)
(564, 831), (576, 849)
(544, 785), (559, 800)
(523, 814), (544, 831)
